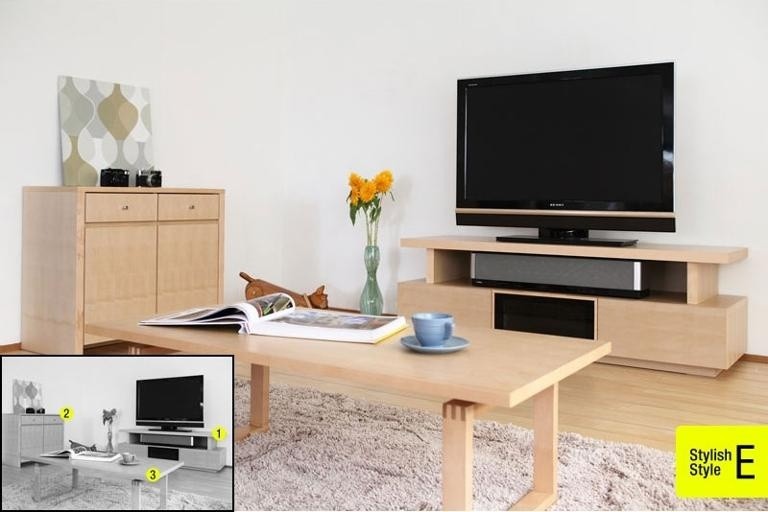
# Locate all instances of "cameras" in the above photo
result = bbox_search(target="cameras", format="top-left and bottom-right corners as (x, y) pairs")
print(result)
(26, 407), (36, 414)
(101, 169), (129, 187)
(136, 170), (162, 187)
(36, 407), (46, 414)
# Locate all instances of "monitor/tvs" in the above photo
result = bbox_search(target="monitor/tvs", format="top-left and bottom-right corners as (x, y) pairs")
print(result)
(134, 373), (205, 432)
(454, 60), (676, 246)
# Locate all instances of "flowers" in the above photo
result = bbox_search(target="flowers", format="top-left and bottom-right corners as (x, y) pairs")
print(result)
(345, 168), (397, 248)
(99, 407), (117, 427)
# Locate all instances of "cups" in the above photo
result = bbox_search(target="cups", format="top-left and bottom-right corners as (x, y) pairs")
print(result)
(122, 452), (136, 463)
(412, 311), (454, 348)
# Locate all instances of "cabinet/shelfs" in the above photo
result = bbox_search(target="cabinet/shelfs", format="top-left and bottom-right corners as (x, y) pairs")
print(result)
(115, 427), (228, 473)
(2, 412), (64, 468)
(18, 184), (226, 356)
(394, 234), (752, 379)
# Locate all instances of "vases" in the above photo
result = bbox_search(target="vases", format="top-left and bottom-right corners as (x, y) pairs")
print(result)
(359, 243), (385, 316)
(107, 431), (113, 455)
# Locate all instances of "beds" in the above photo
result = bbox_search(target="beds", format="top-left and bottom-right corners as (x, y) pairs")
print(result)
(80, 306), (614, 511)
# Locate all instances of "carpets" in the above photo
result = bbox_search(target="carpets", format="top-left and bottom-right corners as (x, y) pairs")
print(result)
(2, 470), (232, 510)
(234, 381), (767, 509)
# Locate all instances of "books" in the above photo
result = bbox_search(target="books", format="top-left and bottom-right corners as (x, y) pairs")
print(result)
(40, 448), (123, 463)
(137, 291), (412, 346)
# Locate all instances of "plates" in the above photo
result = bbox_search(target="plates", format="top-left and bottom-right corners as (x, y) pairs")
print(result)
(400, 333), (471, 354)
(120, 460), (141, 466)
